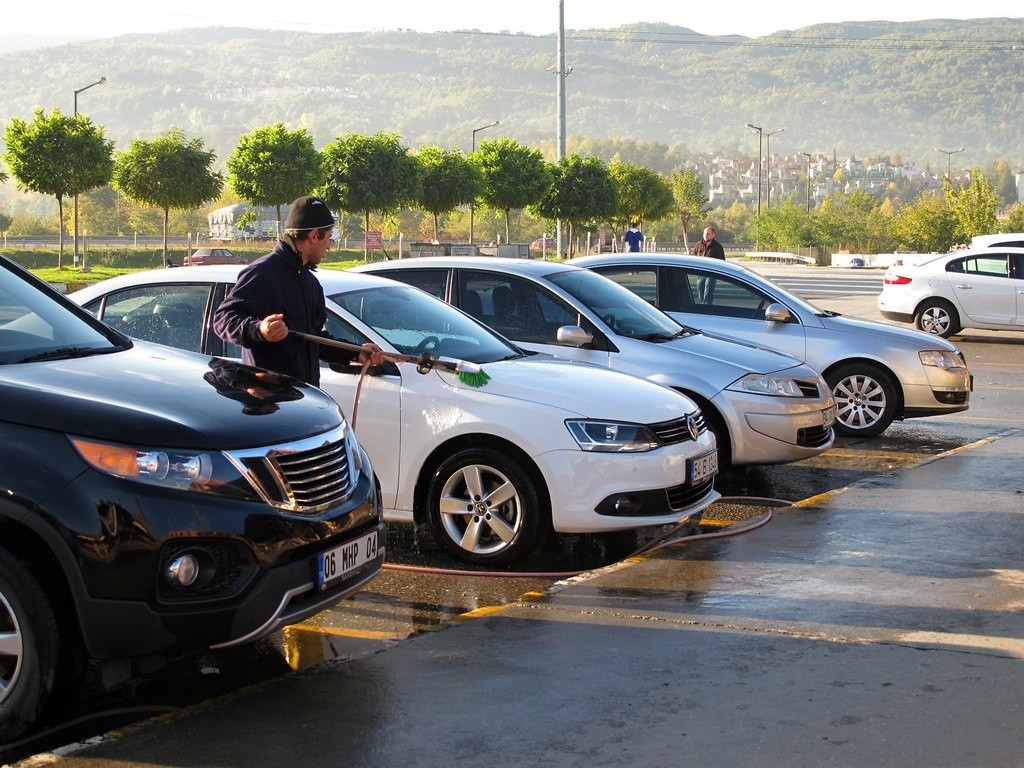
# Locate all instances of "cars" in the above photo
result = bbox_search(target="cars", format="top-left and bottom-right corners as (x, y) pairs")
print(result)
(345, 257), (837, 489)
(877, 247), (1024, 339)
(563, 254), (974, 439)
(0, 265), (721, 569)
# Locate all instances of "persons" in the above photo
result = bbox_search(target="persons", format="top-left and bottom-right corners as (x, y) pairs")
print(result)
(622, 223), (643, 276)
(203, 357), (305, 416)
(211, 195), (335, 392)
(692, 227), (726, 304)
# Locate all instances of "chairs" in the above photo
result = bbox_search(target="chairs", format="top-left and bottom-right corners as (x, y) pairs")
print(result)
(463, 290), (484, 322)
(493, 285), (537, 332)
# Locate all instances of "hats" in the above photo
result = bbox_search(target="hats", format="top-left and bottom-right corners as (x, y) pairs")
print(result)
(285, 196), (335, 230)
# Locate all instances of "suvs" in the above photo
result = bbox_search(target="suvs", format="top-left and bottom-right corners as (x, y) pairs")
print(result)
(0, 255), (384, 743)
(961, 231), (1024, 275)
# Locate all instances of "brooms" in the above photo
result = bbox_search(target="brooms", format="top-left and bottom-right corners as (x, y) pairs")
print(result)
(288, 330), (491, 388)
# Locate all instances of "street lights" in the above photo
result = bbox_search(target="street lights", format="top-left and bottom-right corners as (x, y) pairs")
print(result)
(961, 169), (971, 181)
(752, 128), (786, 210)
(469, 121), (498, 245)
(798, 152), (811, 212)
(74, 77), (111, 266)
(934, 147), (965, 193)
(745, 124), (762, 252)
